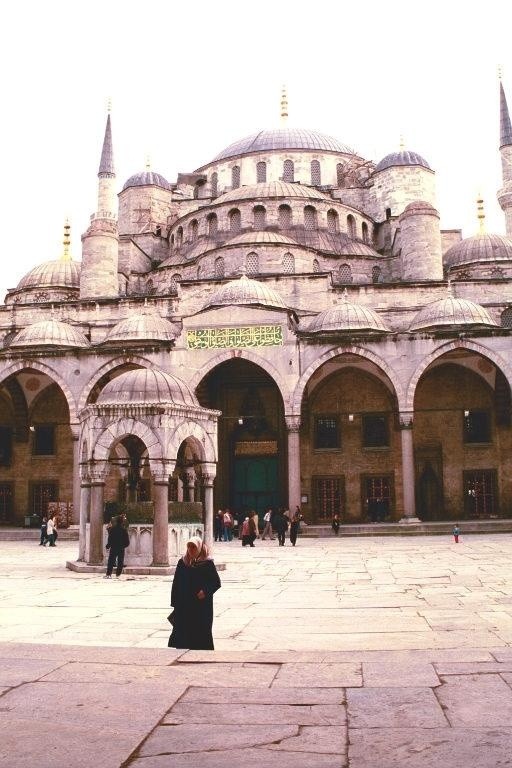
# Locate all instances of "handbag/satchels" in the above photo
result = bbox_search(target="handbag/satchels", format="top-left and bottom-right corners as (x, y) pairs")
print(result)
(167, 611), (176, 628)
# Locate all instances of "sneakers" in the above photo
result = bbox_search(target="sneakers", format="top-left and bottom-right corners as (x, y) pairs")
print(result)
(103, 575), (120, 579)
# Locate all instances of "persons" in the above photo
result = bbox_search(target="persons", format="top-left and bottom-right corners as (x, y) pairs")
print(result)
(167, 536), (221, 650)
(332, 514), (340, 536)
(216, 506), (304, 547)
(106, 513), (129, 567)
(452, 525), (459, 543)
(39, 516), (58, 546)
(103, 517), (129, 579)
